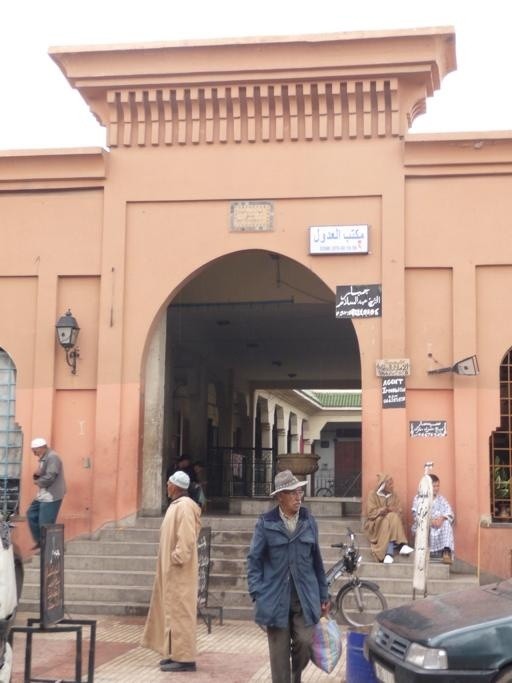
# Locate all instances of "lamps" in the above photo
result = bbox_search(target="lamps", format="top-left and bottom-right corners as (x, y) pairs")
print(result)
(51, 308), (83, 376)
(421, 345), (482, 380)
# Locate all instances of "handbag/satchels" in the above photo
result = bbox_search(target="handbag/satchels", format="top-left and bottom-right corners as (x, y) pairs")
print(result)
(310, 617), (342, 675)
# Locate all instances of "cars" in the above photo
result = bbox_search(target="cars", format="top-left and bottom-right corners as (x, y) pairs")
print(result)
(363, 576), (512, 682)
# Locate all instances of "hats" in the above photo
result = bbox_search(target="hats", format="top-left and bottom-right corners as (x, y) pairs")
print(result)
(31, 438), (45, 448)
(270, 471), (308, 495)
(169, 471), (191, 489)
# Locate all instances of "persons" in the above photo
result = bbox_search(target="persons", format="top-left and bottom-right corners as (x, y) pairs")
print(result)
(26, 436), (69, 554)
(1, 509), (27, 682)
(246, 468), (333, 682)
(167, 453), (201, 511)
(191, 460), (213, 503)
(409, 472), (458, 565)
(139, 469), (204, 673)
(361, 470), (415, 565)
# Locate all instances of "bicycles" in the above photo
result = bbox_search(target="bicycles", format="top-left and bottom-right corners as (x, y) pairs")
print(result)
(315, 477), (362, 496)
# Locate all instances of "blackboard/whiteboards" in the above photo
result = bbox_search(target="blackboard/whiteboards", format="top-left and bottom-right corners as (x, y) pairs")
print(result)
(197, 527), (211, 609)
(40, 523), (64, 632)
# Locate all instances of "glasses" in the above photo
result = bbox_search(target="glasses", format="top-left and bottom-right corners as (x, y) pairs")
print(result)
(289, 491), (305, 497)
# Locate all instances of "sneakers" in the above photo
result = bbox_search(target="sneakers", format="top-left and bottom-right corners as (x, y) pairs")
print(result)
(161, 662), (196, 670)
(160, 659), (180, 664)
(399, 545), (413, 555)
(443, 552), (452, 563)
(384, 555), (393, 564)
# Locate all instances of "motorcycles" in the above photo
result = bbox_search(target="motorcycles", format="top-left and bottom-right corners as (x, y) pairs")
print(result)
(258, 526), (389, 634)
